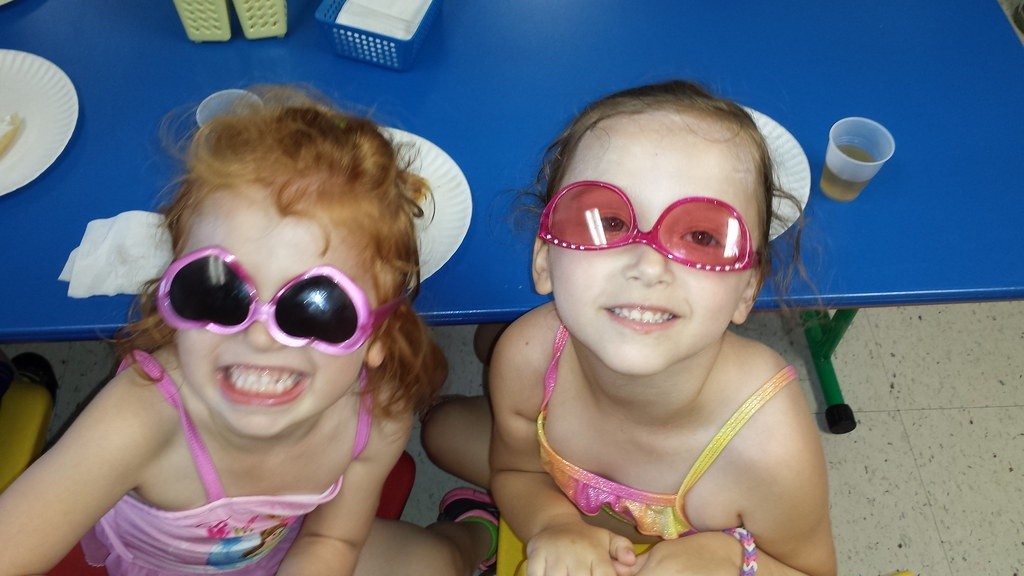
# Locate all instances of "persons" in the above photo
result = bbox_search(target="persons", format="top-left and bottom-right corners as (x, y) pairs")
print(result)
(0, 85), (499, 576)
(421, 78), (838, 576)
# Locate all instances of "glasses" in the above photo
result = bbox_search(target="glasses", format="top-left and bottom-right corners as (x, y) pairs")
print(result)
(156, 247), (405, 357)
(538, 180), (759, 273)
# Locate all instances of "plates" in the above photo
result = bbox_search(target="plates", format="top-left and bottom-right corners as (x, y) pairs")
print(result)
(0, 49), (79, 198)
(740, 104), (811, 241)
(377, 125), (473, 289)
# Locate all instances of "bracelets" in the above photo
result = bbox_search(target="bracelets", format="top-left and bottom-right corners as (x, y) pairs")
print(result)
(723, 525), (758, 576)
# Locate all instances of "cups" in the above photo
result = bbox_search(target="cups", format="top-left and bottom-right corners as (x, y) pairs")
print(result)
(820, 117), (896, 202)
(196, 89), (265, 128)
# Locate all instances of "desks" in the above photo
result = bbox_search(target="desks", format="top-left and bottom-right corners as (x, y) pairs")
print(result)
(0, 0), (1023, 435)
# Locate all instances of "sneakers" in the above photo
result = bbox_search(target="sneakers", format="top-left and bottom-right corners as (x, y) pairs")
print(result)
(436, 487), (502, 576)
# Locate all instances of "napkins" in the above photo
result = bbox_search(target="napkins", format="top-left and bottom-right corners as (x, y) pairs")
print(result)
(334, 0), (432, 63)
